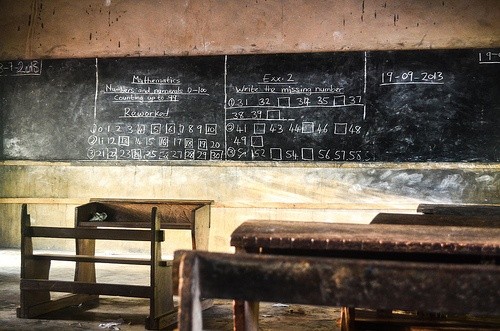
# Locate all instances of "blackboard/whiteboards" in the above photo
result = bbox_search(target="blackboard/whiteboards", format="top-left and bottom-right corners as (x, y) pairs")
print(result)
(0, 45), (500, 170)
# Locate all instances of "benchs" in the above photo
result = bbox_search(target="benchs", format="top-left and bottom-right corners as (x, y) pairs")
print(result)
(16, 204), (178, 331)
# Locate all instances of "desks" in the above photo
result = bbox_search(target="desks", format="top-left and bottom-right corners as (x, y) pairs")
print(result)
(172, 202), (500, 331)
